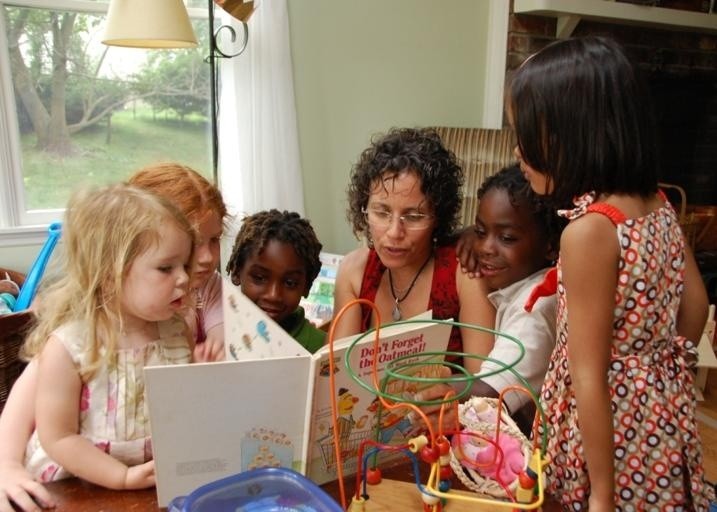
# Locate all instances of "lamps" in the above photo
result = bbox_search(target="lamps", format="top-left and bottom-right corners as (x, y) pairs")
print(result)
(101, 0), (258, 187)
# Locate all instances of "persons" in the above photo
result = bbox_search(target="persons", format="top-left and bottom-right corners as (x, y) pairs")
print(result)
(0, 162), (227, 512)
(407, 162), (560, 448)
(505, 31), (717, 512)
(16, 181), (207, 494)
(222, 209), (334, 358)
(325, 124), (493, 379)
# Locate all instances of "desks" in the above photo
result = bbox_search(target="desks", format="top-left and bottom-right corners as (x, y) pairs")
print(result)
(12, 459), (567, 512)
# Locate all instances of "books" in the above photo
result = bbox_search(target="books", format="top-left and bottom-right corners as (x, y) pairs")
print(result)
(141, 270), (455, 512)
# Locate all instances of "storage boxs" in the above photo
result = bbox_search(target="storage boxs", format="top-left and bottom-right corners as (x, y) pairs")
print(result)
(169, 466), (344, 512)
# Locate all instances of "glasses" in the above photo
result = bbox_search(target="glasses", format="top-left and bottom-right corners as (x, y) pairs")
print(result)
(362, 206), (437, 231)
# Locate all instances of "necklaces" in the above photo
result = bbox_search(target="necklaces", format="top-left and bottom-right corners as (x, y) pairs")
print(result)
(386, 250), (439, 322)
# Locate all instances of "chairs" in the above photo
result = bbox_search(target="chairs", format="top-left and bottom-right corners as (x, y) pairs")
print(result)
(415, 122), (521, 230)
